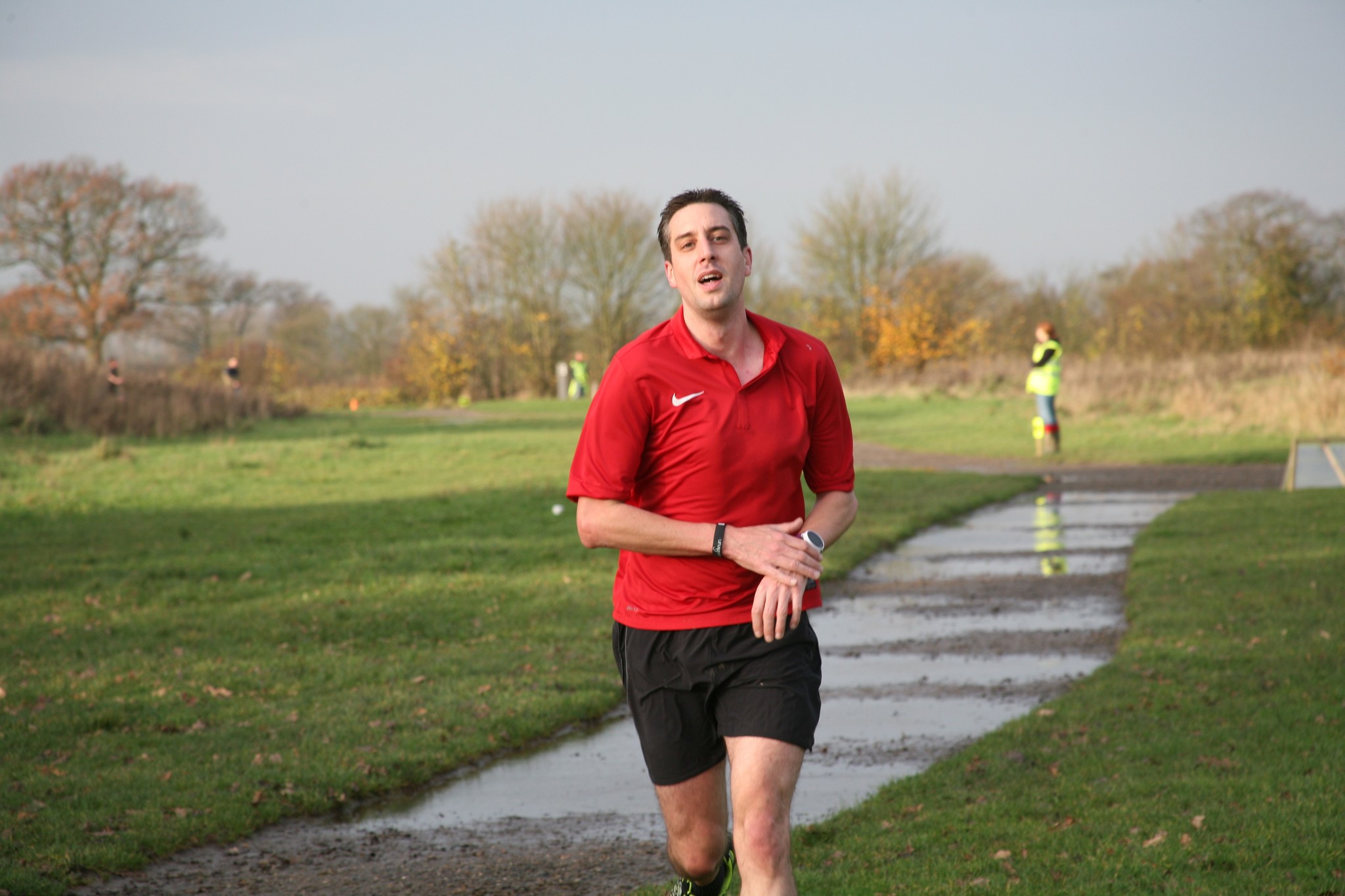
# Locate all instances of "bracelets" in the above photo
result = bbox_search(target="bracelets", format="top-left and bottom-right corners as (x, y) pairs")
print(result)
(712, 523), (726, 558)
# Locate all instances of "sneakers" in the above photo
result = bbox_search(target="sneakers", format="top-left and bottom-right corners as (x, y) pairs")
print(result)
(669, 828), (736, 896)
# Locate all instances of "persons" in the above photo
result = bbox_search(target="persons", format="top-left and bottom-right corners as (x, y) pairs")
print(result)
(1024, 321), (1062, 453)
(567, 188), (860, 896)
(567, 353), (589, 398)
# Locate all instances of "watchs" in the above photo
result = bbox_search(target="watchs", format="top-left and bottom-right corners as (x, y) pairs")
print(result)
(794, 530), (825, 554)
